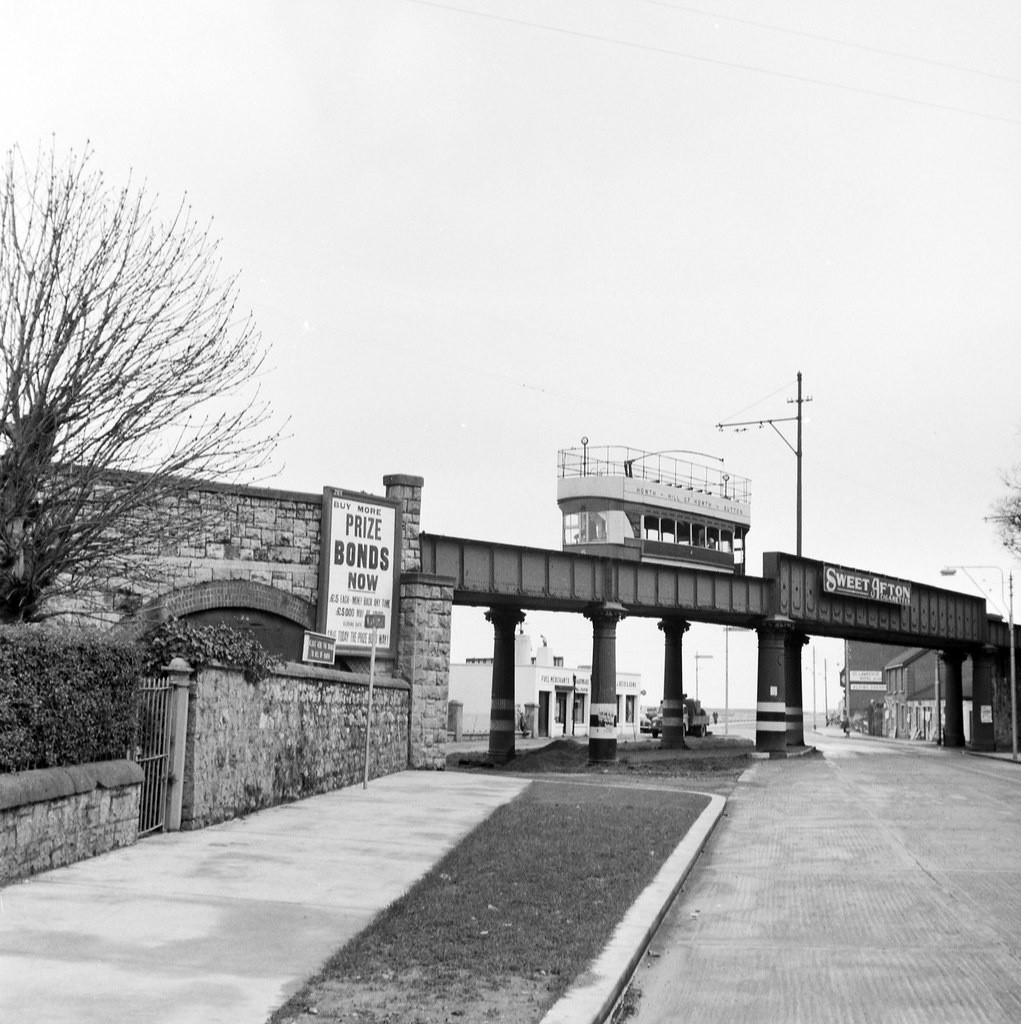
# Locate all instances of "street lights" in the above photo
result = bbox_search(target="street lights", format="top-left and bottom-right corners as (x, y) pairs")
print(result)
(940, 566), (1018, 760)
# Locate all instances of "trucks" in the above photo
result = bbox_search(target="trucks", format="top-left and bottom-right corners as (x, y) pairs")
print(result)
(650, 698), (711, 738)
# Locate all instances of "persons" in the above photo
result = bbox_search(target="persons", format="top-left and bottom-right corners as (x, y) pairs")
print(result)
(844, 717), (850, 738)
